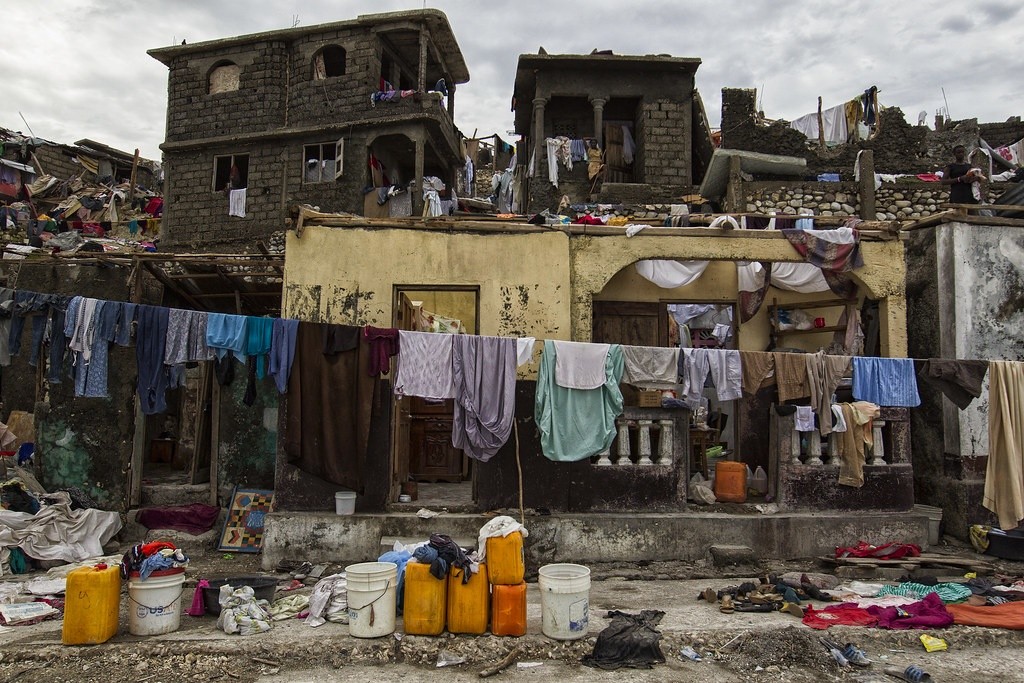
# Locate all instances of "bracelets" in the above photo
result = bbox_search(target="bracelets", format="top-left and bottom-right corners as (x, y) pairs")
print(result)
(958, 176), (960, 182)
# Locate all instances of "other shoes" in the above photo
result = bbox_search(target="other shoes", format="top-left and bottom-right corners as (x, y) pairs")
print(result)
(698, 587), (717, 603)
(718, 573), (802, 613)
(776, 584), (810, 599)
(783, 586), (802, 606)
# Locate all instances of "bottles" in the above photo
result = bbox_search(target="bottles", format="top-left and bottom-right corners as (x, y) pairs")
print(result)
(746, 464), (768, 495)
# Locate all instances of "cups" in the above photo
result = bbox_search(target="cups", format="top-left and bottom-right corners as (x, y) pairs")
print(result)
(814, 318), (825, 328)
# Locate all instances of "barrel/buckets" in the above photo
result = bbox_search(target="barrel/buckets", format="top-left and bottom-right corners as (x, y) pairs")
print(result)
(128, 567), (185, 636)
(346, 561), (398, 636)
(700, 458), (725, 481)
(714, 460), (747, 502)
(62, 563), (121, 645)
(914, 504), (943, 545)
(403, 531), (527, 636)
(335, 491), (357, 515)
(537, 563), (592, 640)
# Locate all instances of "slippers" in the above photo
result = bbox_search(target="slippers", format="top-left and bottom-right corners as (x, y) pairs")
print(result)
(820, 636), (871, 667)
(885, 665), (935, 683)
(719, 594), (736, 614)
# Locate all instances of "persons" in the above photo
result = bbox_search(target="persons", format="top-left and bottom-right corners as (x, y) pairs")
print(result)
(942, 145), (981, 215)
(584, 137), (607, 193)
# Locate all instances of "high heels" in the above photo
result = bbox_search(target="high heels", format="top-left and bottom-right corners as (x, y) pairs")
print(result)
(780, 601), (805, 618)
(800, 573), (833, 602)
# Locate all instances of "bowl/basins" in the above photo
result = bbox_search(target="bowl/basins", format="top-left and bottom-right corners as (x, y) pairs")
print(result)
(835, 385), (854, 403)
(839, 377), (852, 386)
(400, 494), (411, 502)
(699, 446), (723, 459)
(974, 525), (1024, 560)
(202, 577), (280, 614)
